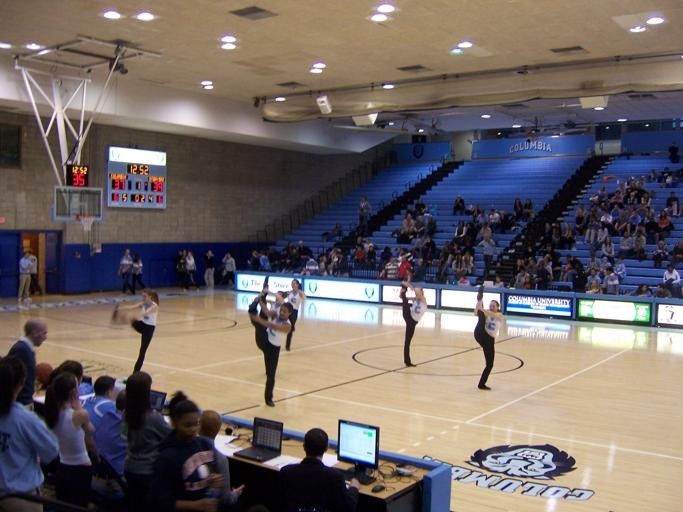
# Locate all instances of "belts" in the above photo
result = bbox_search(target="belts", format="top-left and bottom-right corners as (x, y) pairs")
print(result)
(94, 471), (124, 480)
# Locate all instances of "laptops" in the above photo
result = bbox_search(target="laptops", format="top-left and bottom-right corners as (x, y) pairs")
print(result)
(233, 417), (283, 462)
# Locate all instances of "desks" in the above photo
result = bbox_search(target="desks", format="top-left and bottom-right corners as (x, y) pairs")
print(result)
(163, 410), (451, 512)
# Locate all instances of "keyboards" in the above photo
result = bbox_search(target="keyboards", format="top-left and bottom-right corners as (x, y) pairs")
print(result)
(333, 467), (376, 485)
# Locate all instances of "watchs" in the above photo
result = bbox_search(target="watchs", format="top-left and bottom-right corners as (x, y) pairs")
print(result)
(112, 288), (159, 374)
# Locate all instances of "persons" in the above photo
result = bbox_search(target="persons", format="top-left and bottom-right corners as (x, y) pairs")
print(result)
(17, 252), (33, 302)
(474, 286), (503, 389)
(275, 429), (361, 512)
(248, 297), (293, 407)
(259, 286), (287, 329)
(400, 280), (427, 366)
(285, 279), (306, 351)
(119, 249), (236, 296)
(27, 250), (42, 297)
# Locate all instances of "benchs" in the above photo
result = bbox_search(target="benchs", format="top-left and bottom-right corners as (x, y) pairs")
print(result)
(262, 149), (683, 302)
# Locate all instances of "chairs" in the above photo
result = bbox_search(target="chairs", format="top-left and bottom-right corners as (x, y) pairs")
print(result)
(99, 453), (130, 491)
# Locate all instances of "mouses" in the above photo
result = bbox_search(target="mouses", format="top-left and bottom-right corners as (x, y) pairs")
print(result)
(371, 486), (386, 492)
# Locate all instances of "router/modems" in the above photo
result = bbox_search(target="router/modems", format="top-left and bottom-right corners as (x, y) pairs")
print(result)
(395, 468), (412, 476)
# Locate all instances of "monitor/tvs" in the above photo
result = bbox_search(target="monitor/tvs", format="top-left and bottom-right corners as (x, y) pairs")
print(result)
(337, 420), (379, 475)
(150, 390), (166, 413)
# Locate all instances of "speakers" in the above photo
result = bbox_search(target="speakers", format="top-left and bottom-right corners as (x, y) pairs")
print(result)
(316, 96), (331, 114)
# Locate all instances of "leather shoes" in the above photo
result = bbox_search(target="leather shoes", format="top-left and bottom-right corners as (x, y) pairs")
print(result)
(267, 399), (274, 406)
